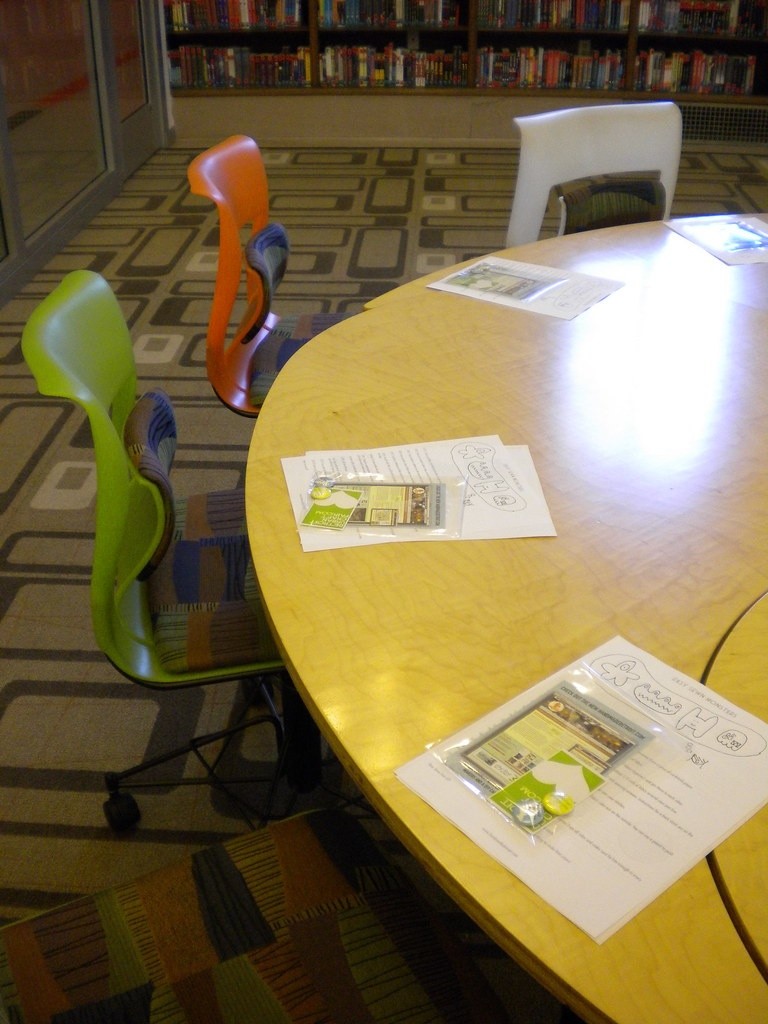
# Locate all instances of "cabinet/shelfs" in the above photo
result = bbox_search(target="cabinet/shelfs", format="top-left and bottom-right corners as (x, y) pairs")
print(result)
(155, 0), (768, 154)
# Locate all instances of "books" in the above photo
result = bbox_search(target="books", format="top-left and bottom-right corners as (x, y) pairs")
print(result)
(164, 0), (768, 37)
(167, 41), (756, 95)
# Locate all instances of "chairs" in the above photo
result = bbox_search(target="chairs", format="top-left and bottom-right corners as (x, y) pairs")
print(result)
(190, 136), (366, 415)
(22, 273), (380, 832)
(506, 100), (686, 251)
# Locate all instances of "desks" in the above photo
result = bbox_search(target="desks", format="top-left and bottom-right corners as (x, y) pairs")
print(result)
(246, 212), (768, 1024)
